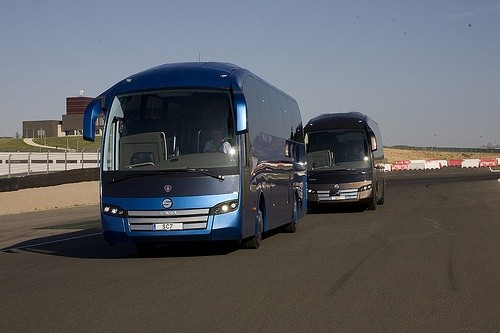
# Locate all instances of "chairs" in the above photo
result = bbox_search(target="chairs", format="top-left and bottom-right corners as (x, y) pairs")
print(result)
(312, 160), (326, 168)
(130, 152), (155, 166)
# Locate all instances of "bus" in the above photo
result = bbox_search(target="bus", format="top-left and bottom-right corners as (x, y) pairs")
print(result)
(299, 110), (385, 212)
(81, 61), (309, 250)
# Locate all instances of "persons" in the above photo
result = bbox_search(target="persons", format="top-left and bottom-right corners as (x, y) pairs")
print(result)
(204, 128), (232, 153)
(352, 147), (360, 158)
